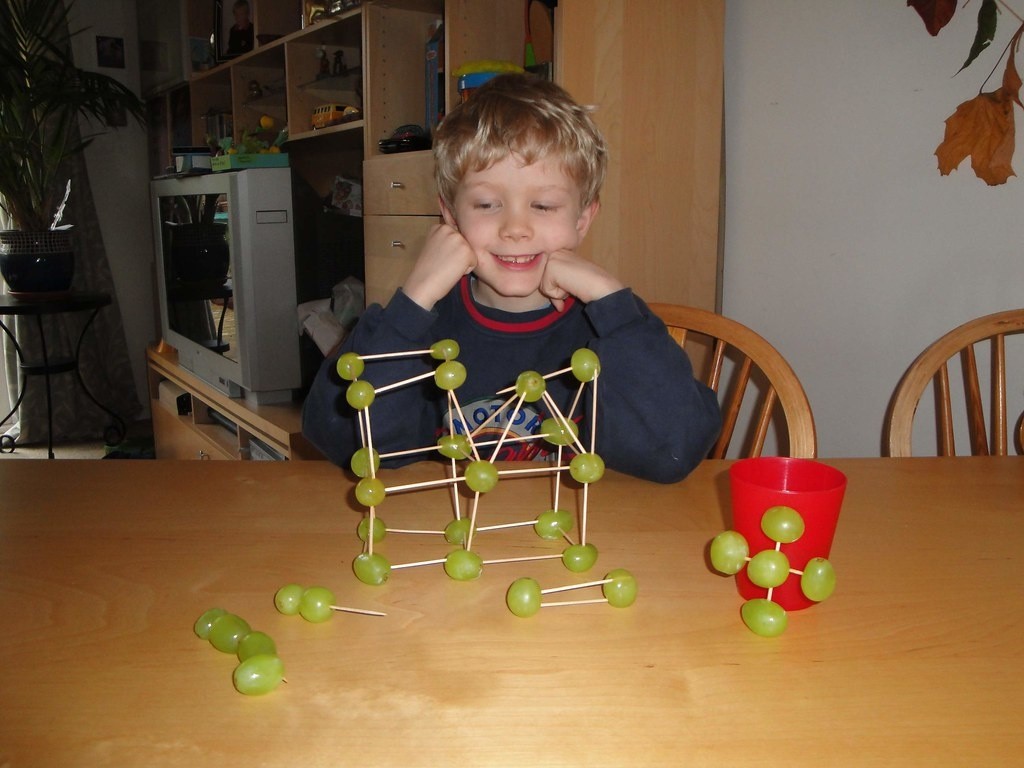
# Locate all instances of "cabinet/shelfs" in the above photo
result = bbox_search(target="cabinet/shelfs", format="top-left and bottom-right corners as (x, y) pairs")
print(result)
(137, 0), (729, 401)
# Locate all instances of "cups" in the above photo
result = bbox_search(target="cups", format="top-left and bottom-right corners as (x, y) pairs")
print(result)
(730, 457), (848, 612)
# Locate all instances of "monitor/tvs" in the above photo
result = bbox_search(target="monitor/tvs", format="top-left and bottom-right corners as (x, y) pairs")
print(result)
(149, 167), (365, 407)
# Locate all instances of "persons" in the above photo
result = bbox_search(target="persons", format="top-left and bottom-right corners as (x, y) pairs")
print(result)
(304, 70), (723, 484)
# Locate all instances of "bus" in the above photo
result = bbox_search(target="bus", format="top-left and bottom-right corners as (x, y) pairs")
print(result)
(310, 104), (348, 130)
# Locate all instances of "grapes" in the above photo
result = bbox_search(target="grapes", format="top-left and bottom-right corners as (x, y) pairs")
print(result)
(192, 340), (836, 697)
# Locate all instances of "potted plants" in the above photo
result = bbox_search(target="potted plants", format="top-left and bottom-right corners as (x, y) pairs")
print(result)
(3, 1), (156, 307)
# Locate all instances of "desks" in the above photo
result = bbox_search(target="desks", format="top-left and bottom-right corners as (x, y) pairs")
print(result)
(0, 293), (131, 467)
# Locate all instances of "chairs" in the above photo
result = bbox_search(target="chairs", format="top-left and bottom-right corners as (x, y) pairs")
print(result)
(885, 310), (1023, 456)
(643, 300), (818, 457)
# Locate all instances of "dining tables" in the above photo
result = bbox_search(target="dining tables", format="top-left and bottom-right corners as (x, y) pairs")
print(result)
(0, 456), (1023, 767)
